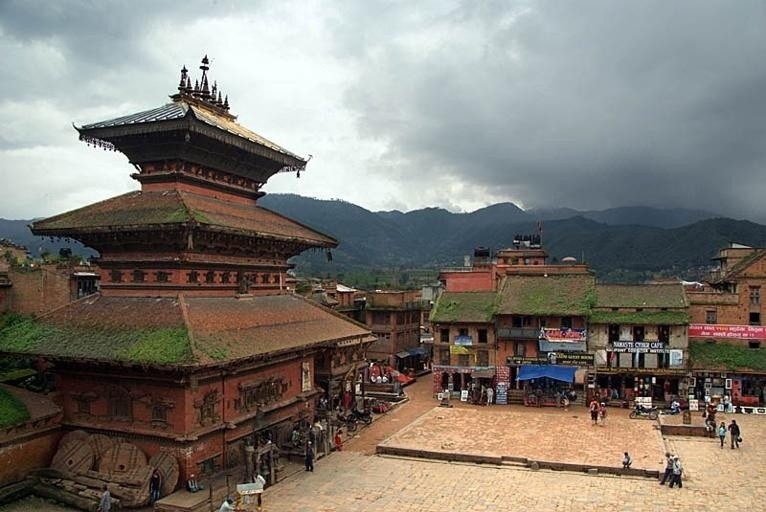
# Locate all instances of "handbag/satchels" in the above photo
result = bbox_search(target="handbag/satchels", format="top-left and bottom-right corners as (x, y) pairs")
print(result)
(737, 436), (742, 443)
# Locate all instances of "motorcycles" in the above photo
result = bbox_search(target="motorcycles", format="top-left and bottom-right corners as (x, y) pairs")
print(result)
(347, 402), (373, 432)
(629, 401), (659, 420)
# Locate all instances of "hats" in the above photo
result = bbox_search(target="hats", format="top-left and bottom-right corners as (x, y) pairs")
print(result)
(673, 455), (679, 459)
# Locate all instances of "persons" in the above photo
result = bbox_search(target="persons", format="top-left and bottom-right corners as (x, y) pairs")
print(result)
(728, 420), (742, 450)
(718, 422), (728, 449)
(147, 469), (161, 508)
(622, 452), (632, 469)
(95, 483), (112, 512)
(667, 456), (682, 489)
(667, 453), (675, 483)
(287, 365), (393, 475)
(658, 451), (673, 486)
(217, 495), (239, 511)
(437, 378), (734, 430)
(252, 469), (265, 508)
(704, 414), (715, 438)
